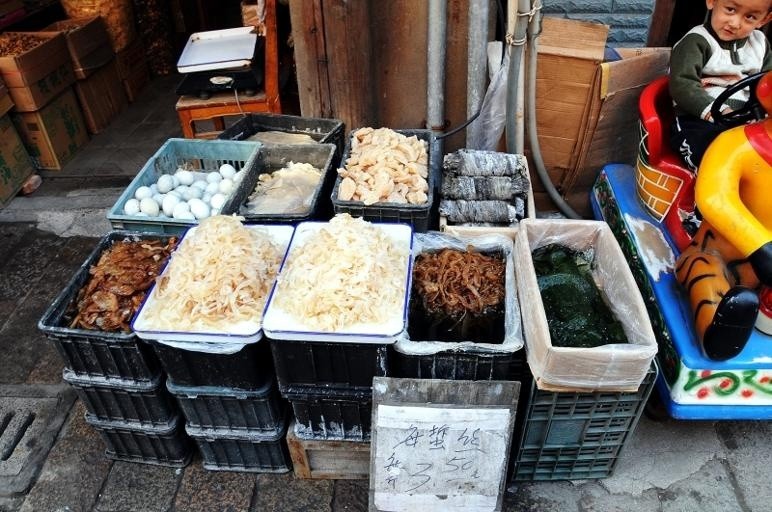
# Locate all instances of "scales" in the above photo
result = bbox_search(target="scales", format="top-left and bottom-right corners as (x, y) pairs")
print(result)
(173, 26), (265, 99)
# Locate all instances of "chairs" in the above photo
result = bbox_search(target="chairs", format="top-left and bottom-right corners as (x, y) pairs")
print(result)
(177, 0), (275, 137)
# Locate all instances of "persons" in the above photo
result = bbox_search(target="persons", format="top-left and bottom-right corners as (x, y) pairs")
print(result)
(666, 0), (772, 238)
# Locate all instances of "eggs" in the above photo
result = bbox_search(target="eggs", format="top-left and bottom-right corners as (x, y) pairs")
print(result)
(125, 163), (242, 220)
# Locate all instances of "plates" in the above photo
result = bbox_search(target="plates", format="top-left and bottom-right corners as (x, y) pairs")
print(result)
(259, 218), (415, 346)
(131, 222), (295, 344)
(176, 24), (259, 75)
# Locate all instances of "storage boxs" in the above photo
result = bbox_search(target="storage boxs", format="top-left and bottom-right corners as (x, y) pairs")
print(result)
(390, 228), (528, 377)
(135, 221), (290, 396)
(0, 121), (33, 211)
(331, 127), (441, 228)
(219, 110), (341, 149)
(439, 152), (535, 228)
(514, 383), (646, 483)
(223, 146), (336, 221)
(286, 434), (367, 481)
(38, 230), (190, 390)
(1, 32), (76, 113)
(11, 90), (93, 172)
(515, 216), (658, 391)
(91, 423), (196, 468)
(293, 399), (371, 440)
(73, 74), (129, 135)
(191, 437), (292, 471)
(109, 140), (265, 228)
(527, 18), (671, 214)
(264, 221), (409, 395)
(78, 388), (175, 433)
(176, 392), (283, 437)
(42, 17), (115, 78)
